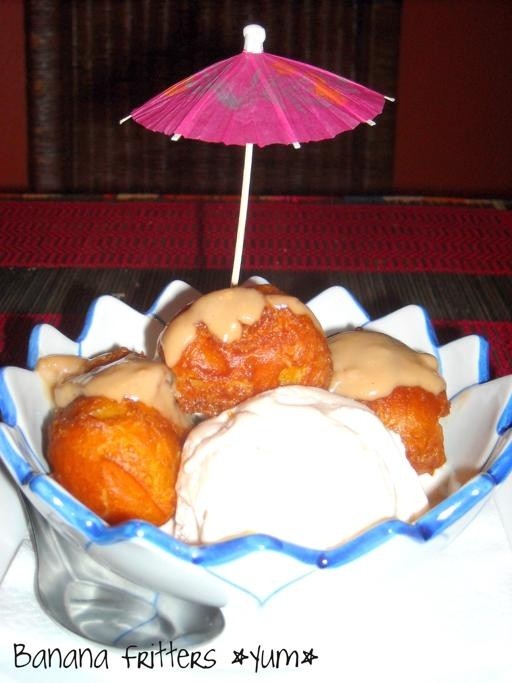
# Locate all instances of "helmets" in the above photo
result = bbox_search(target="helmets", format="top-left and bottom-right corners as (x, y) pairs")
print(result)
(0, 275), (511, 611)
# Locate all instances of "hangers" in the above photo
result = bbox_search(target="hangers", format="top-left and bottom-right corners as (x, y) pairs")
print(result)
(19, 489), (225, 653)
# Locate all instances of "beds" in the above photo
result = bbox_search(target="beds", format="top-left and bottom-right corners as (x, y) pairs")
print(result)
(120, 24), (395, 291)
